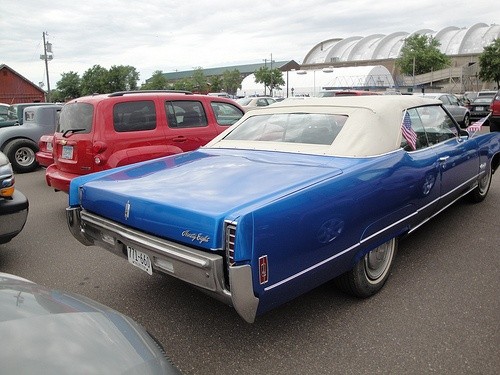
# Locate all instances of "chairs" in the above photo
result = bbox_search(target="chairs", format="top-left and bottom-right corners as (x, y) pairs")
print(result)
(183, 111), (199, 123)
(129, 112), (146, 124)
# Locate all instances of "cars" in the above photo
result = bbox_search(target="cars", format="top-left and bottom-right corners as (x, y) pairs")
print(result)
(0, 152), (28, 244)
(0, 272), (183, 375)
(0, 102), (67, 174)
(205, 90), (500, 133)
(66, 94), (500, 326)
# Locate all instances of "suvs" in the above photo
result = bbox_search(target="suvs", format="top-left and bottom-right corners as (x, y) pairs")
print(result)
(45, 90), (244, 197)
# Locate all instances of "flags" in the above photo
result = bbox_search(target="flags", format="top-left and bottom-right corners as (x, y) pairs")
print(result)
(463, 114), (492, 131)
(401, 111), (417, 151)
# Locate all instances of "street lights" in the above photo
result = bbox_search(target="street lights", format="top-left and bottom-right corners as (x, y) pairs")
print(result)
(410, 49), (417, 90)
(262, 57), (276, 95)
